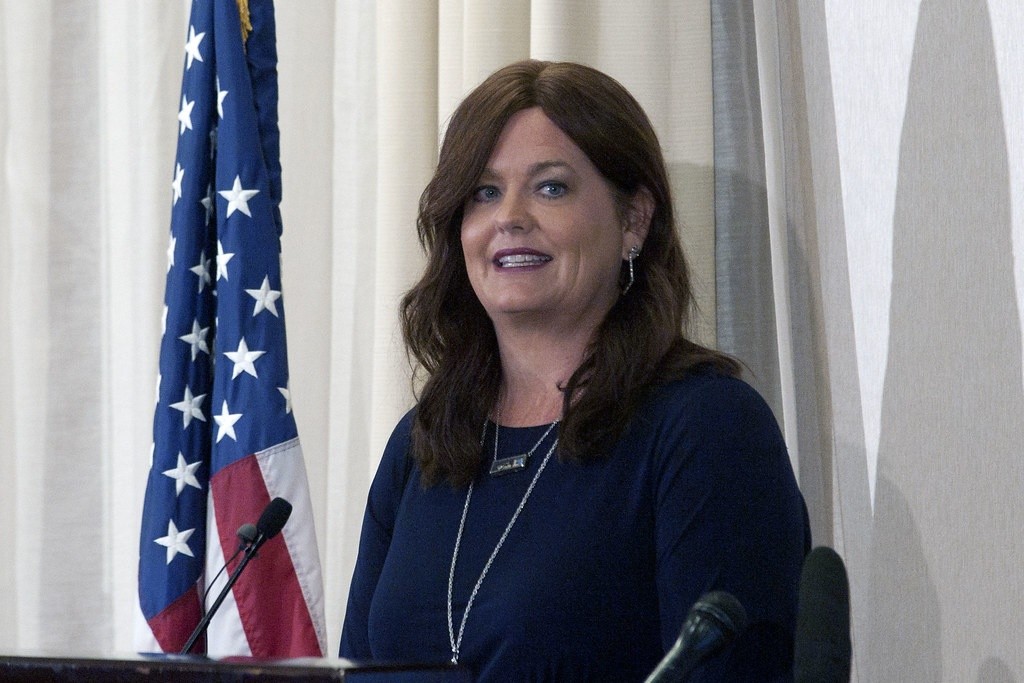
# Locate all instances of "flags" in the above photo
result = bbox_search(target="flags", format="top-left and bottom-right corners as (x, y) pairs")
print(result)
(137, 0), (330, 663)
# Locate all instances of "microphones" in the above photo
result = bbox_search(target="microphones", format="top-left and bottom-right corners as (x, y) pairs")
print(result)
(646, 592), (748, 683)
(202, 524), (257, 657)
(178, 497), (293, 655)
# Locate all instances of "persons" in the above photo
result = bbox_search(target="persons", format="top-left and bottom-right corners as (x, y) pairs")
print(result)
(336, 60), (812, 683)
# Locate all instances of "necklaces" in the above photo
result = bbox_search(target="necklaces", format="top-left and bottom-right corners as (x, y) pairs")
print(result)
(448, 385), (589, 664)
